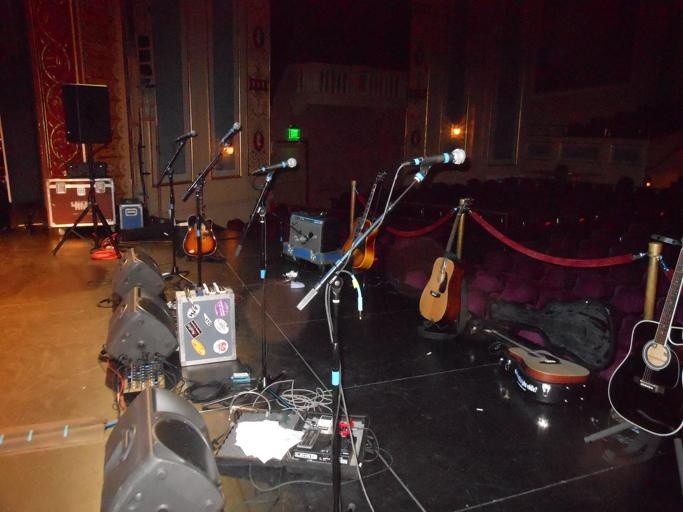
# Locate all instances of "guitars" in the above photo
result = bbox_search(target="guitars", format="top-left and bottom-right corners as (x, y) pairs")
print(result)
(471, 324), (591, 405)
(419, 198), (475, 324)
(342, 168), (389, 271)
(607, 246), (683, 437)
(183, 171), (218, 257)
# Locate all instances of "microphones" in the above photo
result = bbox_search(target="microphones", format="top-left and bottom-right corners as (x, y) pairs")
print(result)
(403, 147), (467, 167)
(251, 157), (298, 175)
(173, 129), (197, 143)
(220, 121), (241, 144)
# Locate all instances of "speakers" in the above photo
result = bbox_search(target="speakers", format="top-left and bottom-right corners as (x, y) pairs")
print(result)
(100, 385), (226, 512)
(105, 286), (179, 366)
(111, 257), (166, 298)
(62, 82), (112, 145)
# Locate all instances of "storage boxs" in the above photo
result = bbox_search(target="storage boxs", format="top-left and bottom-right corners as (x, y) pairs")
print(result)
(118, 204), (143, 230)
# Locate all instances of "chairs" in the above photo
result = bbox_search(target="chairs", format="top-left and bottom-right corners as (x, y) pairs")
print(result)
(226, 165), (682, 382)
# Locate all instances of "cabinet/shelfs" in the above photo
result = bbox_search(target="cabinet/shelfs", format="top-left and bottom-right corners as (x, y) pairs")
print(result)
(45, 178), (116, 236)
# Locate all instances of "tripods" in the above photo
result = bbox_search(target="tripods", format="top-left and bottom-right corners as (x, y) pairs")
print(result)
(52, 144), (123, 261)
(154, 139), (198, 288)
(201, 172), (305, 422)
